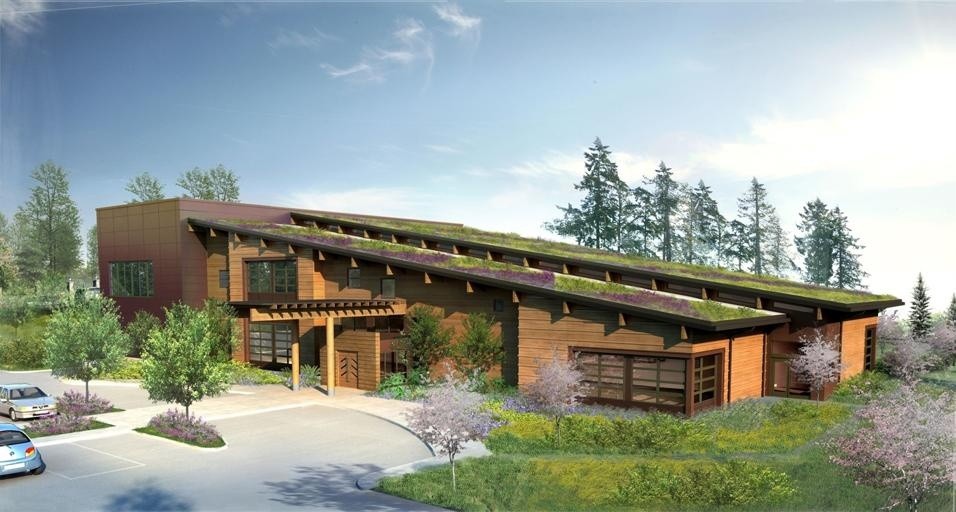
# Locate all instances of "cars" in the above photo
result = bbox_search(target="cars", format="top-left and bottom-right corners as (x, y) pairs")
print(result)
(0, 383), (56, 423)
(0, 422), (43, 480)
